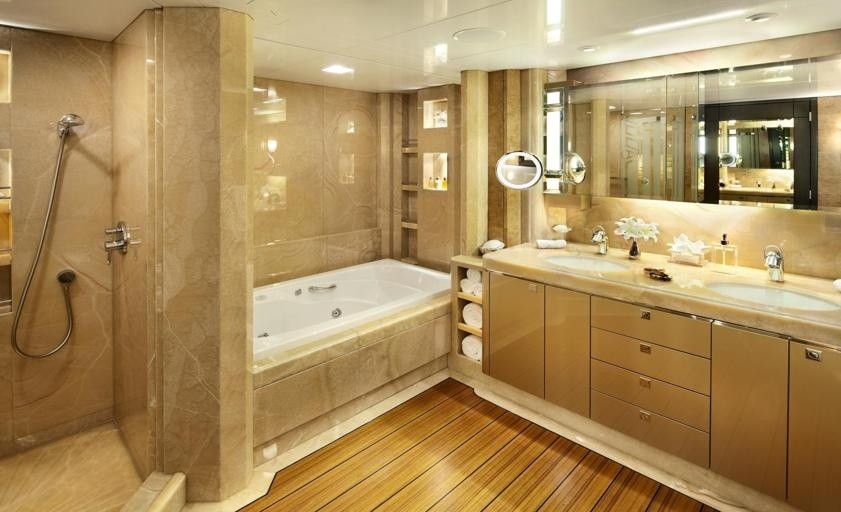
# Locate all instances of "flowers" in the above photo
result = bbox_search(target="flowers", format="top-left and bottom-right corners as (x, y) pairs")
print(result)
(612, 216), (663, 244)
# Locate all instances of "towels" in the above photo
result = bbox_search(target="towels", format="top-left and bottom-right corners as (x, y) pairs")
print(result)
(452, 261), (489, 365)
(537, 239), (567, 248)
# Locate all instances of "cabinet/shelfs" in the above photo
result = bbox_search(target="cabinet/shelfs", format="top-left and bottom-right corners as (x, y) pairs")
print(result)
(711, 318), (788, 502)
(483, 266), (546, 402)
(788, 337), (841, 512)
(591, 293), (711, 491)
(545, 284), (590, 437)
(446, 251), (485, 381)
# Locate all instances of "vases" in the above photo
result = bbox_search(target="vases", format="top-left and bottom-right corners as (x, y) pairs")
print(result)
(628, 241), (641, 260)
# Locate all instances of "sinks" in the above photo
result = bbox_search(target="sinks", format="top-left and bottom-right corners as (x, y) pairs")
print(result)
(704, 281), (841, 312)
(544, 254), (631, 273)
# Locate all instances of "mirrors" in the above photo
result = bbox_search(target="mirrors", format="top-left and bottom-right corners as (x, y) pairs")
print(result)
(494, 149), (547, 191)
(560, 151), (586, 186)
(725, 123), (795, 171)
(558, 50), (841, 213)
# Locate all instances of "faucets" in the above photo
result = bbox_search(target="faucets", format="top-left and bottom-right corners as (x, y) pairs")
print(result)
(592, 229), (606, 255)
(764, 245), (785, 282)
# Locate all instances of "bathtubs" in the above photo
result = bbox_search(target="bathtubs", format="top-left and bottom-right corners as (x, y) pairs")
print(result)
(253, 256), (452, 356)
